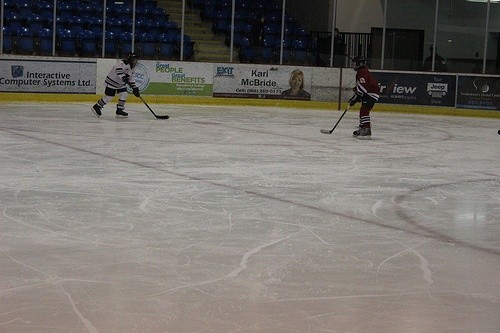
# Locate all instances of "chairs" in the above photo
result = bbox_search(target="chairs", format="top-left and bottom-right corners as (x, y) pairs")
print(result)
(0, 0), (327, 68)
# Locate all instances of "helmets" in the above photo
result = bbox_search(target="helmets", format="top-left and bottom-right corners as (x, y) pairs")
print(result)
(127, 53), (137, 66)
(352, 56), (368, 71)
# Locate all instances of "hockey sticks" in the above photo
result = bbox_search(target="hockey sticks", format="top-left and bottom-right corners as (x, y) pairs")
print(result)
(140, 96), (170, 119)
(319, 104), (350, 134)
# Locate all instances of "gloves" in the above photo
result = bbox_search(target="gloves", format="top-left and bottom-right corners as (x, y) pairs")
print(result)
(133, 87), (140, 97)
(350, 98), (360, 106)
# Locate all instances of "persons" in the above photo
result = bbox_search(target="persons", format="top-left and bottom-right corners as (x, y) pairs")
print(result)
(322, 28), (344, 68)
(423, 45), (447, 72)
(348, 56), (379, 139)
(250, 13), (265, 63)
(280, 69), (311, 100)
(91, 51), (140, 118)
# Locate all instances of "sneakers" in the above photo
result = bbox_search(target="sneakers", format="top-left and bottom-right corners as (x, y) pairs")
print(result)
(91, 104), (101, 118)
(115, 104), (128, 118)
(353, 128), (362, 136)
(357, 128), (371, 139)
